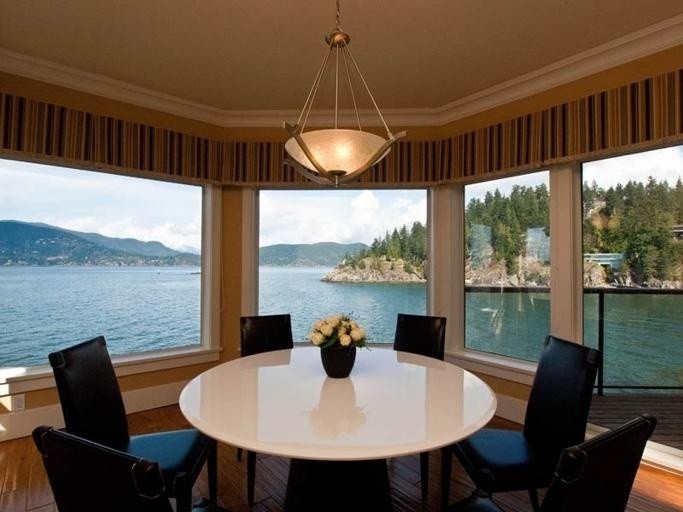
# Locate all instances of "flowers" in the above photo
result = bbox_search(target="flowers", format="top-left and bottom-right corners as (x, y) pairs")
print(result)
(310, 316), (369, 346)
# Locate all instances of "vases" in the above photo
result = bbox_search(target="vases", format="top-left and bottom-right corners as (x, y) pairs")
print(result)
(318, 342), (358, 378)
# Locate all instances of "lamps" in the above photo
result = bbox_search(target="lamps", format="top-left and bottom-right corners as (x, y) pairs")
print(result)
(279, 0), (407, 189)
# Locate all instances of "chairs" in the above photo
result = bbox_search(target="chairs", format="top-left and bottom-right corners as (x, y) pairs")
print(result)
(395, 313), (448, 500)
(52, 335), (215, 512)
(30, 425), (175, 511)
(240, 313), (293, 505)
(448, 335), (598, 504)
(462, 416), (655, 510)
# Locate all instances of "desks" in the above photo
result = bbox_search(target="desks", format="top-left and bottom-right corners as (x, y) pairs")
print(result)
(180, 345), (498, 511)
